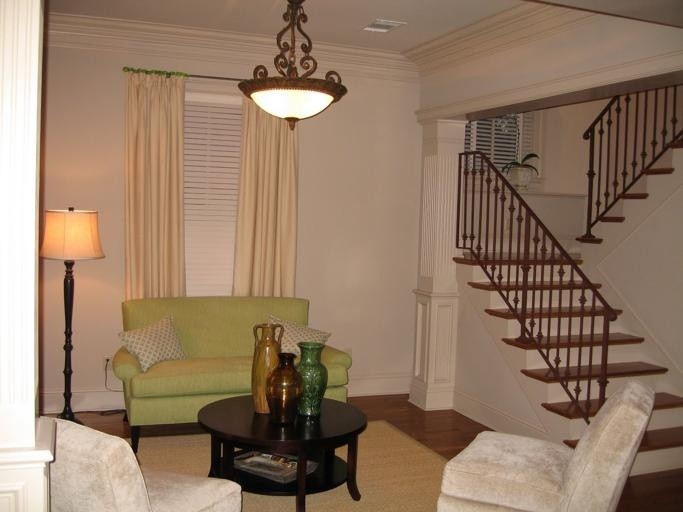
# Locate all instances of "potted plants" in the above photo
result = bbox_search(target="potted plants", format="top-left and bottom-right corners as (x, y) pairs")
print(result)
(502, 153), (540, 191)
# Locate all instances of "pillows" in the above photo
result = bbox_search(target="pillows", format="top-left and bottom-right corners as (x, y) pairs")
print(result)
(268, 315), (330, 358)
(118, 315), (185, 373)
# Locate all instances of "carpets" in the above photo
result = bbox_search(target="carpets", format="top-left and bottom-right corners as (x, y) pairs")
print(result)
(123, 420), (448, 512)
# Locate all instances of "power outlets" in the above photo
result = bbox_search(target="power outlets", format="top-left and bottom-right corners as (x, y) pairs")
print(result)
(103, 356), (113, 369)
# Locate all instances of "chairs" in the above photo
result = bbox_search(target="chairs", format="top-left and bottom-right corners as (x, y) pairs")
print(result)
(33, 418), (242, 512)
(436, 380), (655, 512)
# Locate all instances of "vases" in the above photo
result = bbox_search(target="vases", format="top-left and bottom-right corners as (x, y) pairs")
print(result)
(265, 353), (303, 426)
(253, 323), (284, 415)
(295, 342), (328, 416)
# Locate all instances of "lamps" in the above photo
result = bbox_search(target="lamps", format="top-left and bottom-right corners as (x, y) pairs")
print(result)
(39, 206), (105, 423)
(237, 0), (348, 130)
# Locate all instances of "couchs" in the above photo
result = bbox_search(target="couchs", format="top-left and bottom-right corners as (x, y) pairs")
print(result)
(112, 296), (352, 453)
